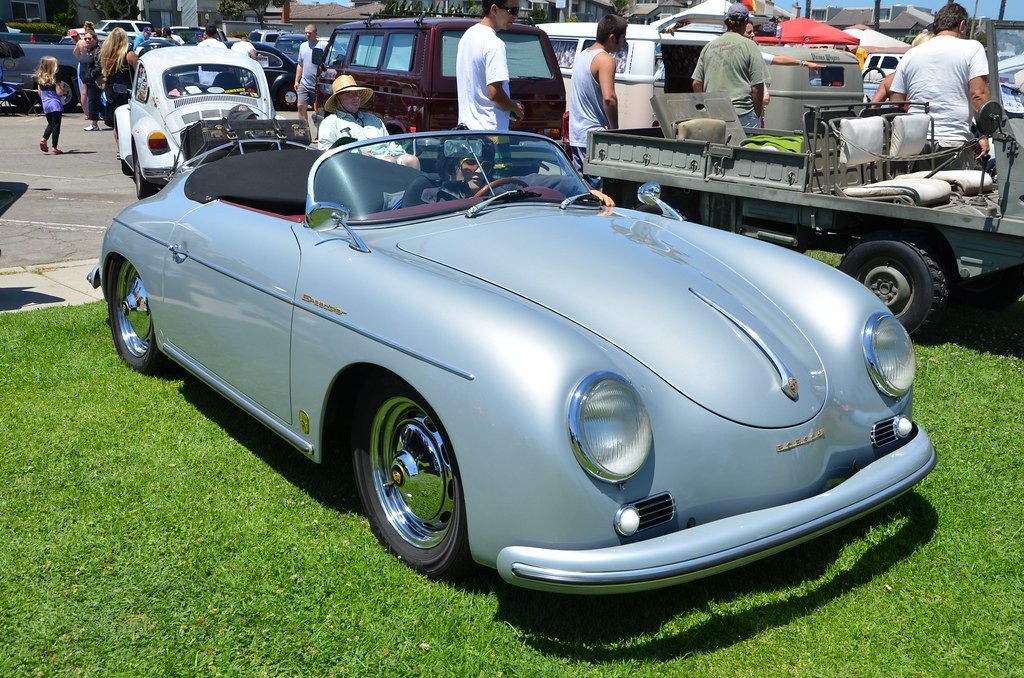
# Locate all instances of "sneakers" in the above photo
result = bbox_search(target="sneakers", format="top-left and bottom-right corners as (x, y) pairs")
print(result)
(83, 125), (99, 131)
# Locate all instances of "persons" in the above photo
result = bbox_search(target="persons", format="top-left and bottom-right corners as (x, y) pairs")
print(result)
(227, 104), (259, 120)
(691, 2), (827, 128)
(420, 123), (615, 207)
(78, 21), (146, 159)
(870, 31), (935, 112)
(318, 75), (421, 170)
(457, 0), (525, 180)
(569, 13), (628, 191)
(294, 24), (328, 143)
(890, 3), (990, 170)
(134, 24), (257, 61)
(70, 30), (81, 43)
(38, 56), (64, 155)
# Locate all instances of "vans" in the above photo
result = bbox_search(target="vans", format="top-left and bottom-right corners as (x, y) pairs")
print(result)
(314, 9), (1024, 147)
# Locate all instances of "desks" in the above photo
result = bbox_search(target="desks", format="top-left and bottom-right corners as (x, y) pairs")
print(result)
(22, 88), (40, 117)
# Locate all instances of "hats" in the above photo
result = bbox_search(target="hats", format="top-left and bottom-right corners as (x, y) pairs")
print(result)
(69, 30), (78, 36)
(144, 27), (152, 34)
(324, 75), (373, 113)
(724, 3), (749, 21)
(194, 31), (203, 37)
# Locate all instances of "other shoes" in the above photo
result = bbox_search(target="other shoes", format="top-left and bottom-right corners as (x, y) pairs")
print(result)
(117, 152), (120, 159)
(40, 140), (49, 152)
(84, 116), (89, 119)
(52, 149), (61, 155)
(99, 116), (103, 120)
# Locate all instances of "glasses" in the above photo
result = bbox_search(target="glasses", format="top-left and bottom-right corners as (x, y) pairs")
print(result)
(84, 37), (91, 40)
(459, 157), (492, 176)
(145, 31), (151, 35)
(499, 5), (519, 16)
(745, 29), (752, 35)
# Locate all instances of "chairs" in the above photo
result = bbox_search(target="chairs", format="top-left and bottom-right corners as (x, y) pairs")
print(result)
(164, 76), (184, 93)
(213, 73), (240, 91)
(890, 110), (993, 197)
(839, 115), (950, 205)
(403, 172), (440, 209)
(0, 62), (24, 117)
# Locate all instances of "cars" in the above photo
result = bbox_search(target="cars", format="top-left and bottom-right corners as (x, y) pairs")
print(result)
(87, 119), (938, 595)
(0, 20), (346, 201)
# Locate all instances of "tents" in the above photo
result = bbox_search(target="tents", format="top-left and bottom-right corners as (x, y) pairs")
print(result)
(650, 1), (781, 36)
(751, 18), (911, 53)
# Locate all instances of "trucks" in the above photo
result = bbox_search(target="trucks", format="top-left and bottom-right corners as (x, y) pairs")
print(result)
(584, 20), (1024, 337)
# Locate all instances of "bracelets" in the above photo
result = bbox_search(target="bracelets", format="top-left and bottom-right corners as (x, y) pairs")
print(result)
(801, 59), (805, 66)
